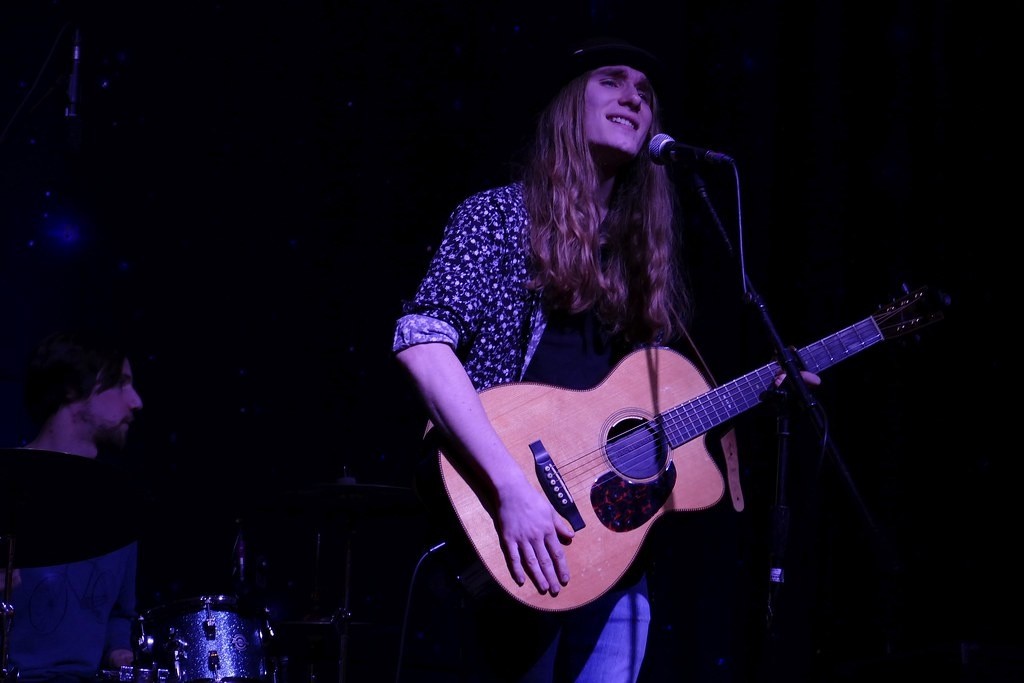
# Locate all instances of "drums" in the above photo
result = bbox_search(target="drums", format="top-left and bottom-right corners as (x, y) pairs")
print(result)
(128, 593), (274, 682)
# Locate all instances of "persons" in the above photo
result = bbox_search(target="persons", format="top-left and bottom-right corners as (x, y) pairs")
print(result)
(391, 28), (821, 683)
(0, 324), (145, 683)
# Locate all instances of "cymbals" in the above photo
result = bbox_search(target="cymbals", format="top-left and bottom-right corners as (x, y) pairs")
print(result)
(0, 446), (149, 570)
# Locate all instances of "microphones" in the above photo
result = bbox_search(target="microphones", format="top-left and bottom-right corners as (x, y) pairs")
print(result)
(649, 134), (735, 170)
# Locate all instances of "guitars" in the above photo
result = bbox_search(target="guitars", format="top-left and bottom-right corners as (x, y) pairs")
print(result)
(422, 282), (953, 615)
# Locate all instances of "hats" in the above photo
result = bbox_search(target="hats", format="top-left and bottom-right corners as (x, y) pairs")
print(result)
(542, 36), (674, 104)
